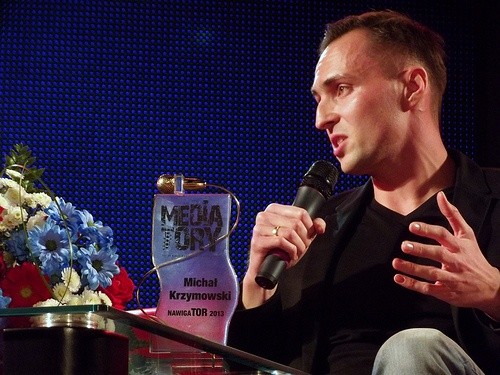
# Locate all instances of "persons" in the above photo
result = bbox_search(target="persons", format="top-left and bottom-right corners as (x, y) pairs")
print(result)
(225, 12), (500, 375)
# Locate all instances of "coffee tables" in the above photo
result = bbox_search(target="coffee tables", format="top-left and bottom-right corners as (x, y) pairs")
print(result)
(0, 304), (306, 375)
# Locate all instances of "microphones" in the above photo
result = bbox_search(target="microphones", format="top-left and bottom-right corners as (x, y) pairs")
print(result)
(254, 160), (340, 290)
(157, 174), (207, 194)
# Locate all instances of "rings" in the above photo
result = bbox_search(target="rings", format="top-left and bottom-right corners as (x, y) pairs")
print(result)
(272, 226), (280, 235)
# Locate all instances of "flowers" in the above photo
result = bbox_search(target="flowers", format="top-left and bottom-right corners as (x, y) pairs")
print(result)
(0, 142), (135, 332)
